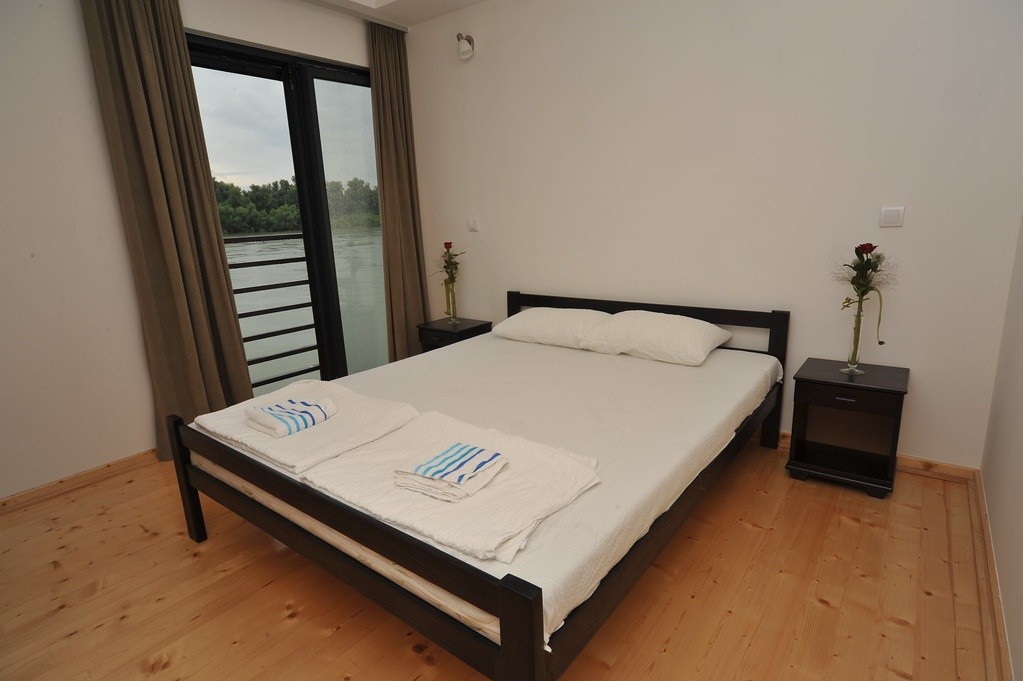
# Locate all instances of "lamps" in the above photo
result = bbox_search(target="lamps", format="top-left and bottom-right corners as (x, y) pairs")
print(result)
(457, 33), (474, 60)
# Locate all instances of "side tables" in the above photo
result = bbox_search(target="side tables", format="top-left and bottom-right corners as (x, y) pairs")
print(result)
(784, 357), (910, 499)
(415, 316), (492, 353)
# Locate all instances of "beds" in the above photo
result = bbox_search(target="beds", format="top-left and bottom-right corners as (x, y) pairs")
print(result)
(165, 292), (792, 681)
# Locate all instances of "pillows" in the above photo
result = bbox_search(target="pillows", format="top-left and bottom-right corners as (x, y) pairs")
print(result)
(492, 306), (732, 367)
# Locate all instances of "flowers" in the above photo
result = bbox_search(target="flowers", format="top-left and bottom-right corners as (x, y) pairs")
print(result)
(436, 240), (465, 317)
(828, 242), (899, 363)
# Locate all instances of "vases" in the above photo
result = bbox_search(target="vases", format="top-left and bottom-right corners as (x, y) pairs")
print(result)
(839, 308), (866, 376)
(445, 279), (461, 325)
